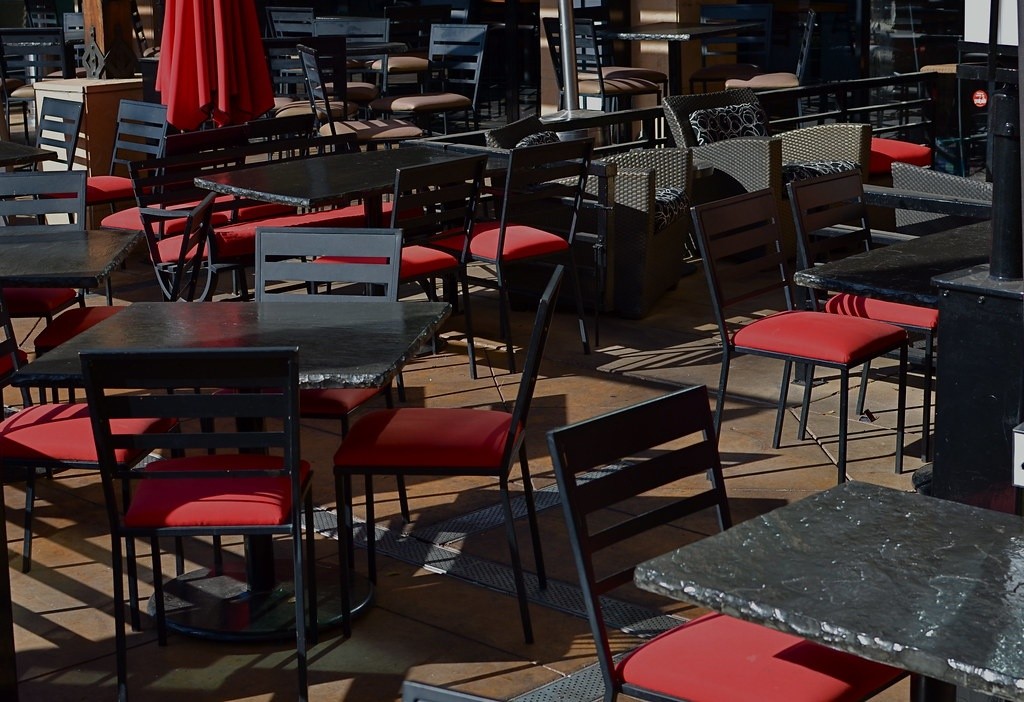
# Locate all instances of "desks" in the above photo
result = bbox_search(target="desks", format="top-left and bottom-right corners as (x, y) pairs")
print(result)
(0, 226), (143, 482)
(794, 220), (994, 492)
(0, 140), (58, 169)
(586, 21), (758, 149)
(634, 480), (1024, 702)
(291, 42), (409, 125)
(9, 298), (452, 647)
(193, 146), (509, 358)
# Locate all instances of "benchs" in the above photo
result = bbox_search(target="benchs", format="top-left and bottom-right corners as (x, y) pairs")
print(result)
(129, 133), (425, 304)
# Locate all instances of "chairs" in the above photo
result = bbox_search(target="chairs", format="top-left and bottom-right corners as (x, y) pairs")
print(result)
(0, 0), (1024, 702)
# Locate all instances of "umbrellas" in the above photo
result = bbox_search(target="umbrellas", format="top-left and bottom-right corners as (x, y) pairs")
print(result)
(154, 0), (276, 132)
